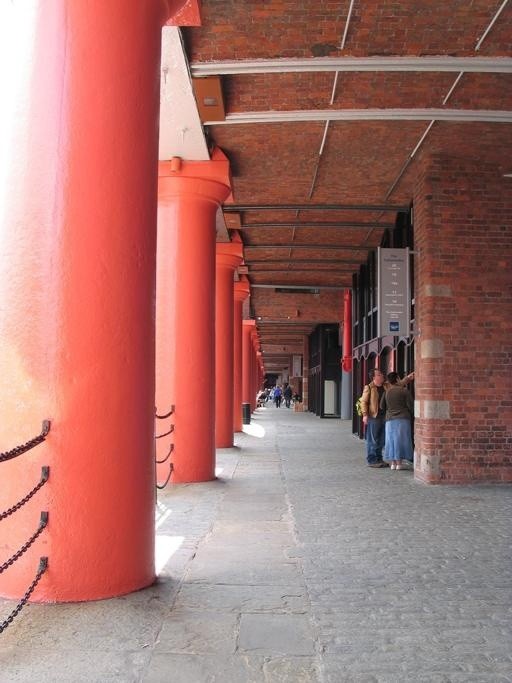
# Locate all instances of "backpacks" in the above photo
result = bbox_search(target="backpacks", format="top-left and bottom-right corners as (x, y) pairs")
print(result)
(356, 384), (371, 417)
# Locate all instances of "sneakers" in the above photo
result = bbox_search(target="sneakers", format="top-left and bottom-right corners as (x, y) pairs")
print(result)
(391, 463), (410, 470)
(367, 461), (389, 468)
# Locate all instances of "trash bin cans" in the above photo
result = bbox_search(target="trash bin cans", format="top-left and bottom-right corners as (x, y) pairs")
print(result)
(242, 403), (250, 424)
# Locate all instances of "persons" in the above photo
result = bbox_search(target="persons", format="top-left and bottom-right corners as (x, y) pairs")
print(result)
(379, 371), (413, 472)
(360, 367), (415, 469)
(255, 382), (300, 409)
